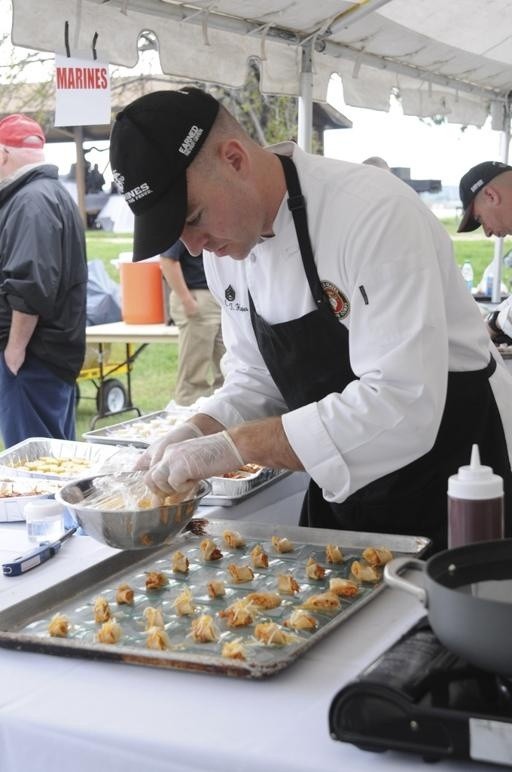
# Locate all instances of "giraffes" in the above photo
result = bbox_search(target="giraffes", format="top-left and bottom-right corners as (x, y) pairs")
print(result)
(325, 612), (510, 767)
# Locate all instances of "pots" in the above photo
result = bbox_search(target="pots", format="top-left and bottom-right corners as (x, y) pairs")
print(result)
(382, 539), (512, 680)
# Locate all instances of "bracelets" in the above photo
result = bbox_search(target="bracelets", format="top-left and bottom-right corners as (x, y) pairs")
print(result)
(54, 472), (212, 552)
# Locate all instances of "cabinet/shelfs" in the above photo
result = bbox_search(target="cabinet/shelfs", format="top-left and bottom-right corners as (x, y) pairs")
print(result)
(445, 444), (507, 551)
(460, 259), (474, 291)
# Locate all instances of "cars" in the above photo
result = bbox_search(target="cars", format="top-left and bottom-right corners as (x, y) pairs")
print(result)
(23, 499), (64, 548)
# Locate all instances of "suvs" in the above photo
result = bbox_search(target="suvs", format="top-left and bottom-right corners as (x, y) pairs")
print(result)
(111, 251), (163, 323)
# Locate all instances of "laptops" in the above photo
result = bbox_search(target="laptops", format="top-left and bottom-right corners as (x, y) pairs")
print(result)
(12, 454), (89, 475)
(103, 416), (185, 441)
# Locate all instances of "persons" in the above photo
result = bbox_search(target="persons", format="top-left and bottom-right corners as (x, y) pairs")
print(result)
(362, 156), (390, 172)
(106, 85), (512, 568)
(456, 160), (512, 350)
(67, 157), (118, 231)
(0, 114), (88, 448)
(158, 239), (225, 407)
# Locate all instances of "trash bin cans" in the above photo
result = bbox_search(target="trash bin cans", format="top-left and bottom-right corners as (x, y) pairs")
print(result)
(487, 311), (502, 332)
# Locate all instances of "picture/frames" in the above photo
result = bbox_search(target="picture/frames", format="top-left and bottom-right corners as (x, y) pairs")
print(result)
(84, 322), (182, 431)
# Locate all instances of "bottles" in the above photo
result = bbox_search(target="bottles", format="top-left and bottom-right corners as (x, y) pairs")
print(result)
(457, 161), (511, 232)
(0, 114), (45, 148)
(110, 87), (220, 263)
